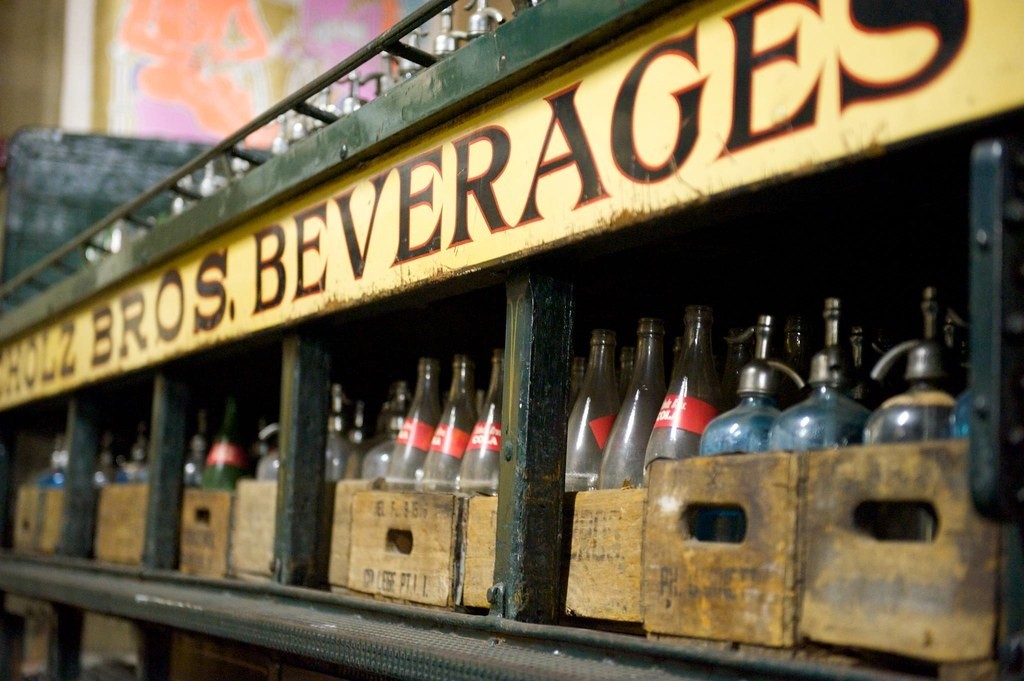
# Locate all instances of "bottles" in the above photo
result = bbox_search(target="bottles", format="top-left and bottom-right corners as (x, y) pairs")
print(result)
(322, 348), (503, 483)
(566, 287), (971, 541)
(95, 414), (155, 484)
(182, 389), (281, 491)
(30, 431), (70, 492)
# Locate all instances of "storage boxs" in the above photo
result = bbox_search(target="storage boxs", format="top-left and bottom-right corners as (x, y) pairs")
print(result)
(8, 436), (1018, 681)
